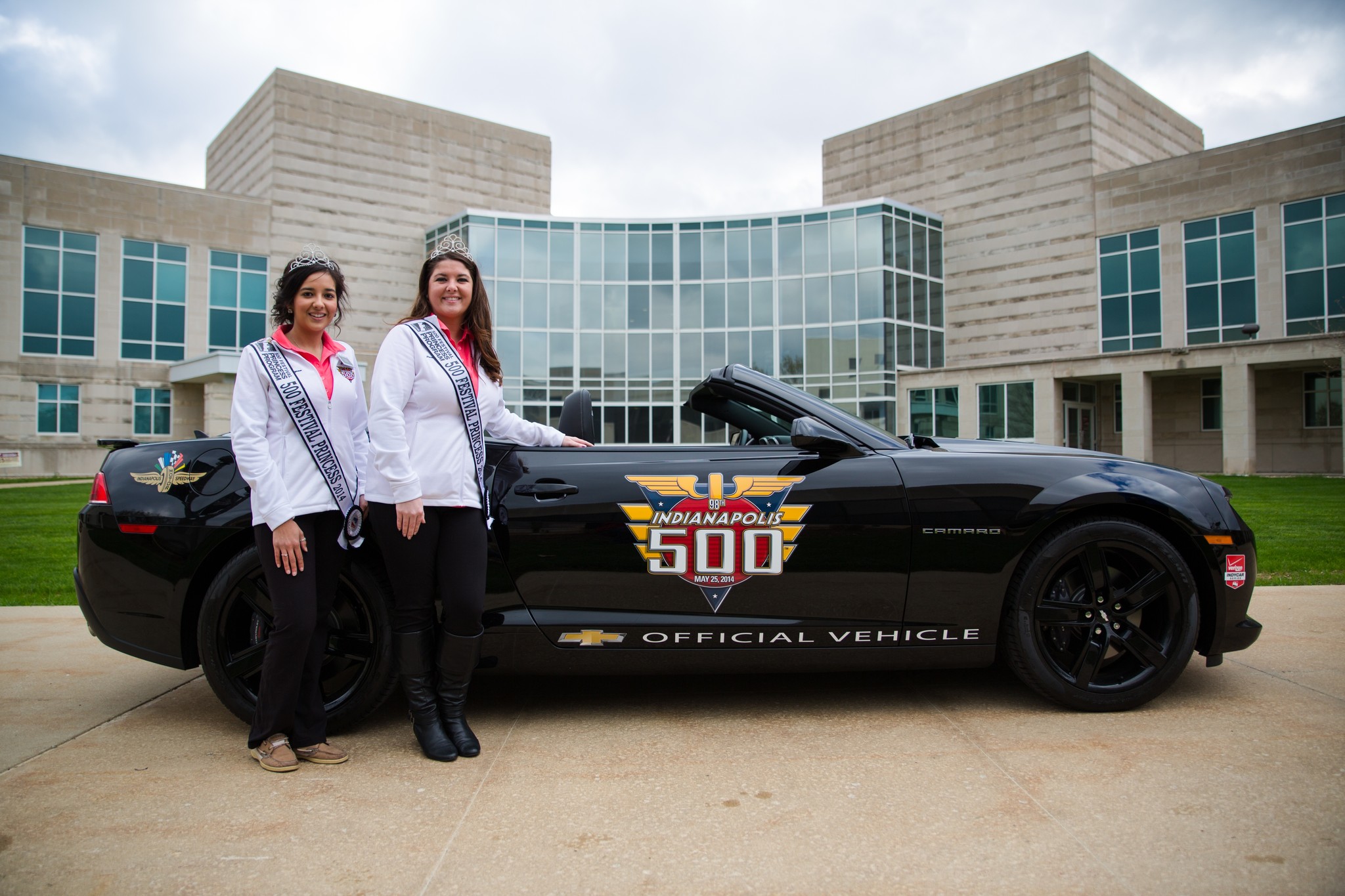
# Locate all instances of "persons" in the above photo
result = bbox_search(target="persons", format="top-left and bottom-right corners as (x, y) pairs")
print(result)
(363, 248), (595, 762)
(229, 255), (371, 775)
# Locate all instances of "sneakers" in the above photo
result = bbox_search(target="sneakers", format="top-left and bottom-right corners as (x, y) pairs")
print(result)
(251, 733), (299, 771)
(292, 742), (348, 764)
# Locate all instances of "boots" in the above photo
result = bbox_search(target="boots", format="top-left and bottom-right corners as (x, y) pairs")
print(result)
(439, 623), (485, 757)
(396, 625), (458, 761)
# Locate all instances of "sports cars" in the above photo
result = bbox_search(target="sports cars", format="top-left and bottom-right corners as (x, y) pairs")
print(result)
(70, 361), (1267, 739)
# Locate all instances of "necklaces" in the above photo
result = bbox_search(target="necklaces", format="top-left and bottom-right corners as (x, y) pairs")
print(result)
(289, 331), (322, 364)
